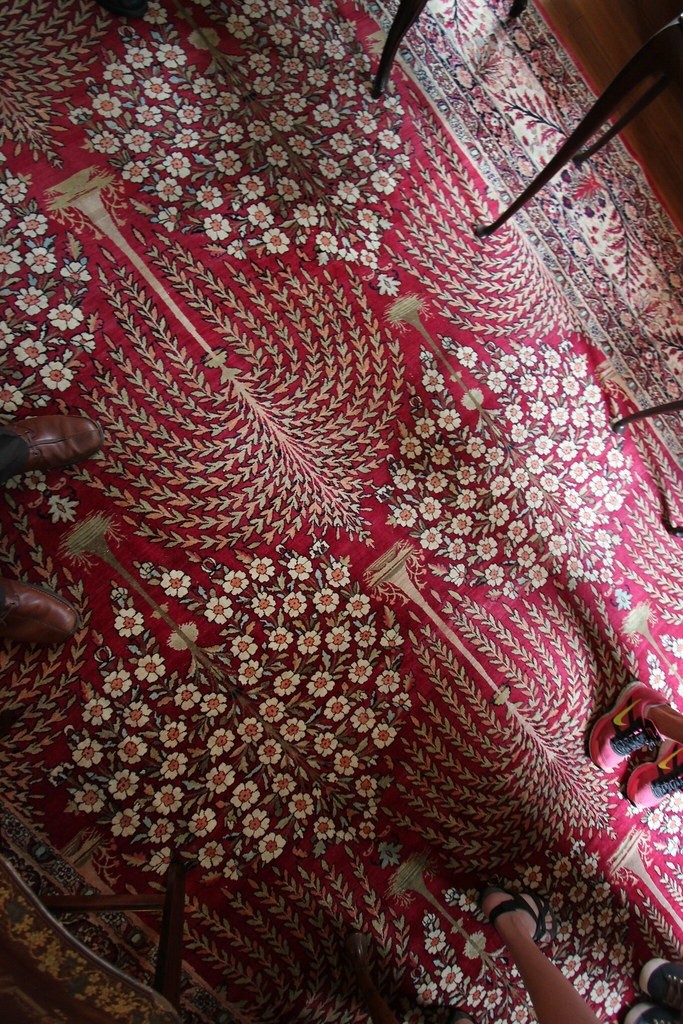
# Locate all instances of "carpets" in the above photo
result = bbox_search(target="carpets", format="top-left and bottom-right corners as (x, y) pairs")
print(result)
(0, 0), (683, 1024)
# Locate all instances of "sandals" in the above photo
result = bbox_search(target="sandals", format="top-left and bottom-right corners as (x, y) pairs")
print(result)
(446, 1011), (477, 1024)
(476, 887), (557, 943)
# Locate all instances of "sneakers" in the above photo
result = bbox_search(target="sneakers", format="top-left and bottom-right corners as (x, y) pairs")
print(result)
(627, 738), (683, 810)
(589, 681), (672, 774)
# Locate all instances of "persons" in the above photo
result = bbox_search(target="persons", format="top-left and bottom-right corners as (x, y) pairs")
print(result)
(0, 414), (105, 643)
(448, 886), (602, 1024)
(589, 681), (683, 811)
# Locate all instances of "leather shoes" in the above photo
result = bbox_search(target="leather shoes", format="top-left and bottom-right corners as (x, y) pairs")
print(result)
(0, 576), (80, 645)
(0, 415), (105, 476)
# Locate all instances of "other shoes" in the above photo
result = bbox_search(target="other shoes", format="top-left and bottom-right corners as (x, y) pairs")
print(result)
(623, 1001), (683, 1024)
(638, 957), (683, 1019)
